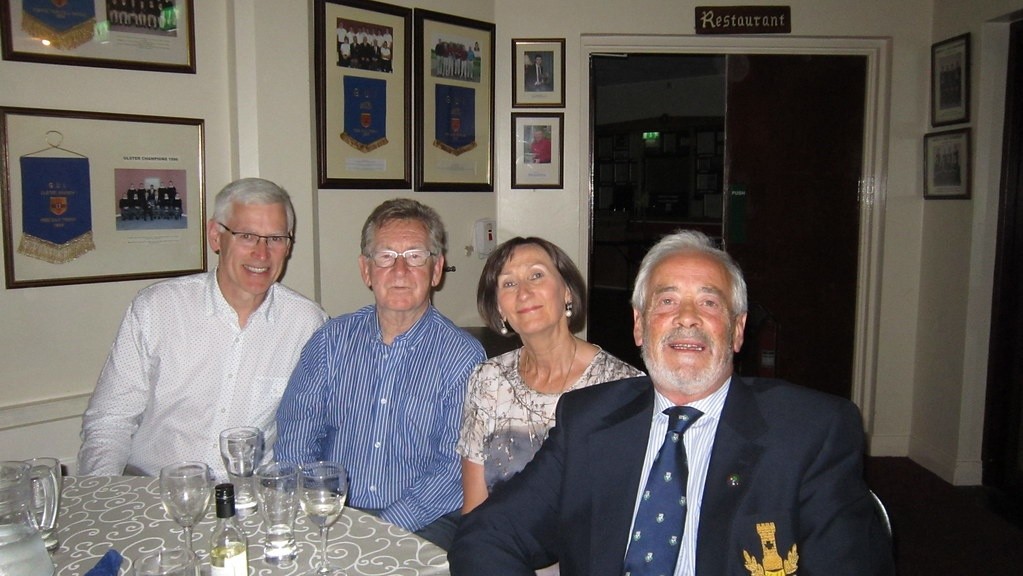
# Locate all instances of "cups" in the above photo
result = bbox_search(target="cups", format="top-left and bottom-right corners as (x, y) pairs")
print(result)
(253, 461), (301, 565)
(0, 460), (59, 576)
(23, 456), (63, 552)
(219, 426), (263, 516)
(132, 548), (201, 576)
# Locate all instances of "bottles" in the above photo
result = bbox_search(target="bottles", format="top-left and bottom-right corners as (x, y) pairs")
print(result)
(209, 483), (248, 576)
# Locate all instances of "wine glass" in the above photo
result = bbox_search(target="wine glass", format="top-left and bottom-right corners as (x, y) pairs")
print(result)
(298, 461), (349, 576)
(160, 460), (211, 547)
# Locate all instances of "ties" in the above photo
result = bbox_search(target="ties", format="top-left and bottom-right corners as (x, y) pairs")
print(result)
(619, 405), (705, 576)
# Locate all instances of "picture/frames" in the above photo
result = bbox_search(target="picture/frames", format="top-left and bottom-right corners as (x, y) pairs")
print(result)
(931, 32), (970, 128)
(923, 127), (972, 200)
(511, 112), (564, 189)
(314, 0), (412, 189)
(511, 38), (566, 108)
(0, 0), (196, 74)
(0, 106), (207, 289)
(414, 7), (497, 193)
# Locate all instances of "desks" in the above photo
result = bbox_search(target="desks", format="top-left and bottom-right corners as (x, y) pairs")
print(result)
(0, 476), (451, 576)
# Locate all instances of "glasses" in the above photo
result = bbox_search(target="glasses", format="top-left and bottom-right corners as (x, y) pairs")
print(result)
(217, 221), (295, 251)
(363, 247), (438, 268)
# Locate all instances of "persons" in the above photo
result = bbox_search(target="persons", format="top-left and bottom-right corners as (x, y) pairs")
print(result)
(526, 54), (544, 91)
(447, 229), (897, 576)
(109, 0), (174, 31)
(273, 198), (488, 551)
(530, 129), (551, 163)
(77, 178), (331, 478)
(454, 236), (648, 515)
(336, 22), (393, 73)
(435, 38), (480, 81)
(118, 180), (183, 221)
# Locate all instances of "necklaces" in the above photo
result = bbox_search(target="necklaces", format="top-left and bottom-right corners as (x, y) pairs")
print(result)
(525, 335), (577, 446)
